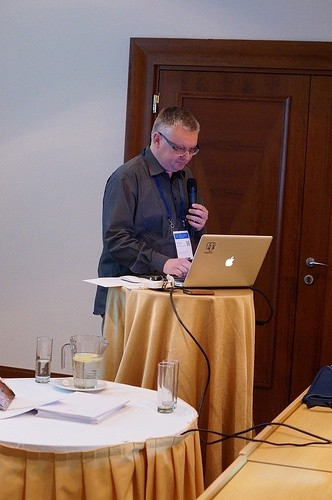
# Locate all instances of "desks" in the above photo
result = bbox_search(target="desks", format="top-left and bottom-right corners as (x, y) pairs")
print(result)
(104, 279), (254, 491)
(0, 378), (205, 500)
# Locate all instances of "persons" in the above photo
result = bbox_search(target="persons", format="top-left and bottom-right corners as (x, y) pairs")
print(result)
(93, 106), (209, 338)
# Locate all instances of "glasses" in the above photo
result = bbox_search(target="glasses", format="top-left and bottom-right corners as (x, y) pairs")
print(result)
(158, 132), (200, 155)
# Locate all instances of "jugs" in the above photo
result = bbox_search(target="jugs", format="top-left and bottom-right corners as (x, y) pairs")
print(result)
(61, 335), (110, 388)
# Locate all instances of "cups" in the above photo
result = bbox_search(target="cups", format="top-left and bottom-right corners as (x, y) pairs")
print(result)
(35, 335), (53, 384)
(157, 362), (175, 414)
(162, 360), (178, 404)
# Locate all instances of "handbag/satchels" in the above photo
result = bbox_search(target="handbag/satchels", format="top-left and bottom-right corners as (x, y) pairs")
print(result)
(302, 365), (332, 409)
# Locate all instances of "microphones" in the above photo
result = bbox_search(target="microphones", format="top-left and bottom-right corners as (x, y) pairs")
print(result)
(186, 177), (198, 229)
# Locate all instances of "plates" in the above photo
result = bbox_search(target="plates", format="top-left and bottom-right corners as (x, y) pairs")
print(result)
(54, 378), (109, 392)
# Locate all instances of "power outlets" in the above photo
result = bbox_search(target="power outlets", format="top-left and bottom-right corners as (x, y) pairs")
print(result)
(146, 274), (177, 291)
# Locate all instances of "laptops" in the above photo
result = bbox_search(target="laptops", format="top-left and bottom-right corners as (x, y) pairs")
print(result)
(166, 234), (273, 288)
